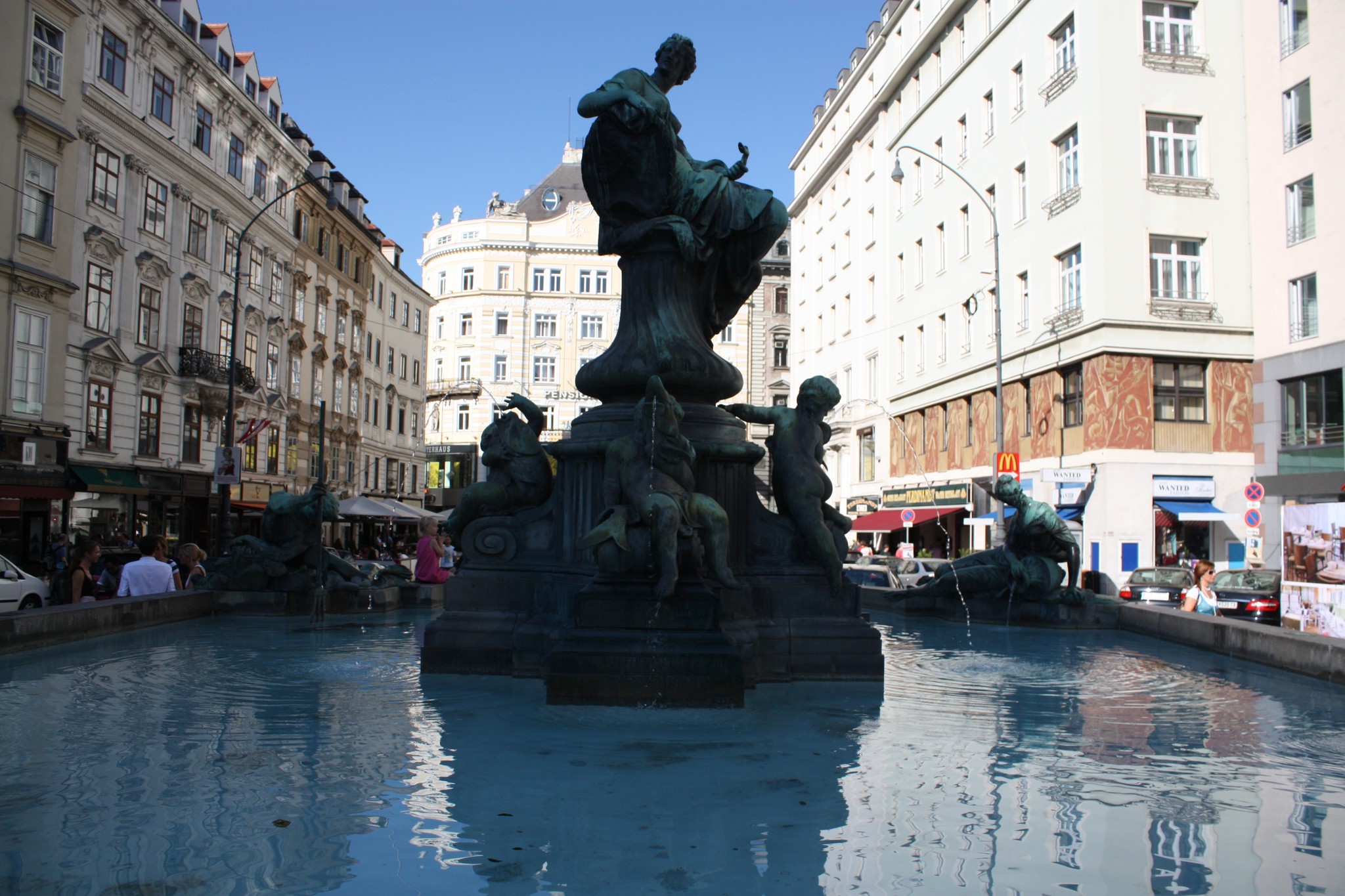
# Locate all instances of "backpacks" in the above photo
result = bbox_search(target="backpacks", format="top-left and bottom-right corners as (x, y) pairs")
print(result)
(43, 544), (65, 572)
(52, 569), (86, 606)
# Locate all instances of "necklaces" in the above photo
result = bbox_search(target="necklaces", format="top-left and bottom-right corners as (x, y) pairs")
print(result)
(1200, 584), (1217, 617)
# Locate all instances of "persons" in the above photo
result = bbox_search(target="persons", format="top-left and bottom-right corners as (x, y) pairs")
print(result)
(437, 392), (552, 535)
(1179, 558), (1224, 619)
(44, 526), (462, 605)
(717, 375), (853, 598)
(577, 33), (789, 336)
(217, 448), (236, 476)
(604, 374), (741, 599)
(847, 537), (944, 564)
(880, 472), (1080, 604)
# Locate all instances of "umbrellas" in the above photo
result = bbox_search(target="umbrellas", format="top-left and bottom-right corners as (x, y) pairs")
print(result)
(337, 495), (459, 520)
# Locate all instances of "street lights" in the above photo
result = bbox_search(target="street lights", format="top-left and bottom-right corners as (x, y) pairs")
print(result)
(891, 145), (1006, 552)
(216, 174), (337, 561)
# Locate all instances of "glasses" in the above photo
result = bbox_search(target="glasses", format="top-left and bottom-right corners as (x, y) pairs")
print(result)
(91, 551), (101, 555)
(156, 547), (164, 550)
(1207, 571), (1216, 574)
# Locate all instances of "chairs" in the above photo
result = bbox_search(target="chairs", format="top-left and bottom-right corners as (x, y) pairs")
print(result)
(1137, 576), (1154, 584)
(1171, 577), (1190, 584)
(1283, 523), (1345, 585)
(1224, 580), (1239, 588)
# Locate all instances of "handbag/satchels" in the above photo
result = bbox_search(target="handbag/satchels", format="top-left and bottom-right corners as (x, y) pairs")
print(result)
(1174, 597), (1197, 612)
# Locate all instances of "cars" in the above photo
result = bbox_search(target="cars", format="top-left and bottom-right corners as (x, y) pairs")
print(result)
(0, 553), (52, 614)
(1118, 567), (1197, 610)
(896, 558), (953, 590)
(843, 564), (905, 589)
(1208, 568), (1282, 627)
(63, 546), (148, 601)
(842, 549), (863, 564)
(854, 555), (905, 578)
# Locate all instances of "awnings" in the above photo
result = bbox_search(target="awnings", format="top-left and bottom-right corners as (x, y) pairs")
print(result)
(68, 465), (147, 496)
(963, 505), (1081, 525)
(1154, 501), (1235, 522)
(848, 507), (964, 534)
